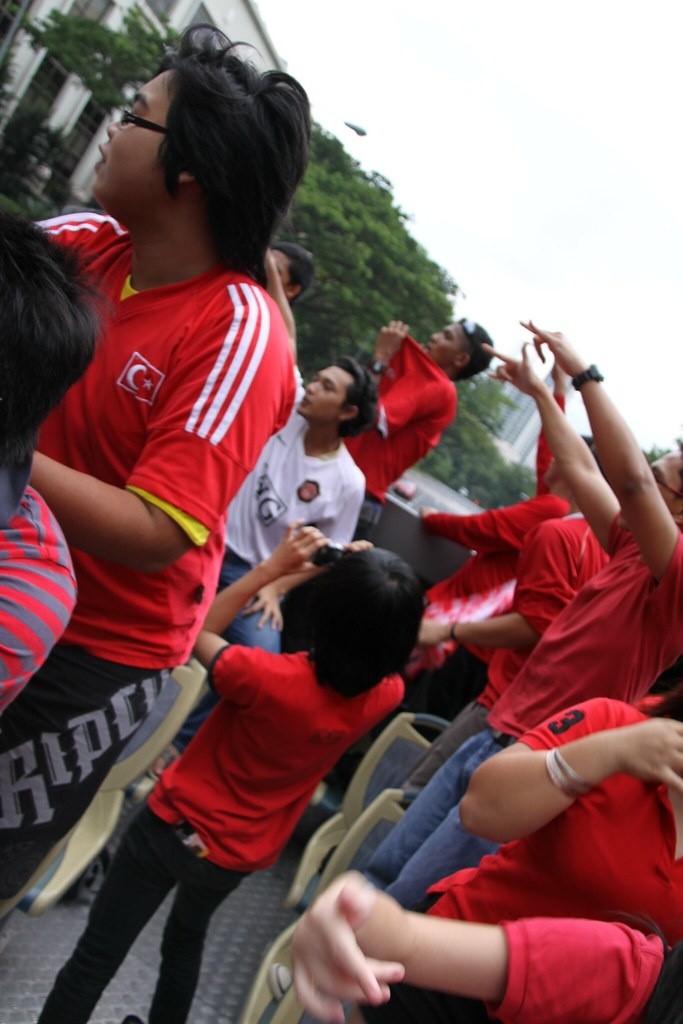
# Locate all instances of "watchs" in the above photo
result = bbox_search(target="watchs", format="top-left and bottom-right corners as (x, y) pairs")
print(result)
(367, 359), (388, 376)
(571, 363), (605, 389)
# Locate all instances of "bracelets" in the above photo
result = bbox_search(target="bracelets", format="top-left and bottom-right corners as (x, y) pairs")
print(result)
(545, 748), (600, 799)
(450, 620), (456, 640)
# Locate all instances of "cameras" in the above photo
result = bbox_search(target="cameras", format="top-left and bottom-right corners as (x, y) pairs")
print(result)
(312, 540), (348, 568)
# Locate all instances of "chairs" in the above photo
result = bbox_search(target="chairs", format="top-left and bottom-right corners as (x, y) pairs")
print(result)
(242, 788), (419, 1024)
(0, 655), (208, 953)
(308, 779), (343, 815)
(281, 712), (454, 914)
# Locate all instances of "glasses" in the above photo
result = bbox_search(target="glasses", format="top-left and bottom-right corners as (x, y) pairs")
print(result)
(458, 318), (474, 340)
(119, 108), (168, 134)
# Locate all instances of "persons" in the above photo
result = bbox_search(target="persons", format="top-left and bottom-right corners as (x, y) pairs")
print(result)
(0, 19), (682, 1024)
